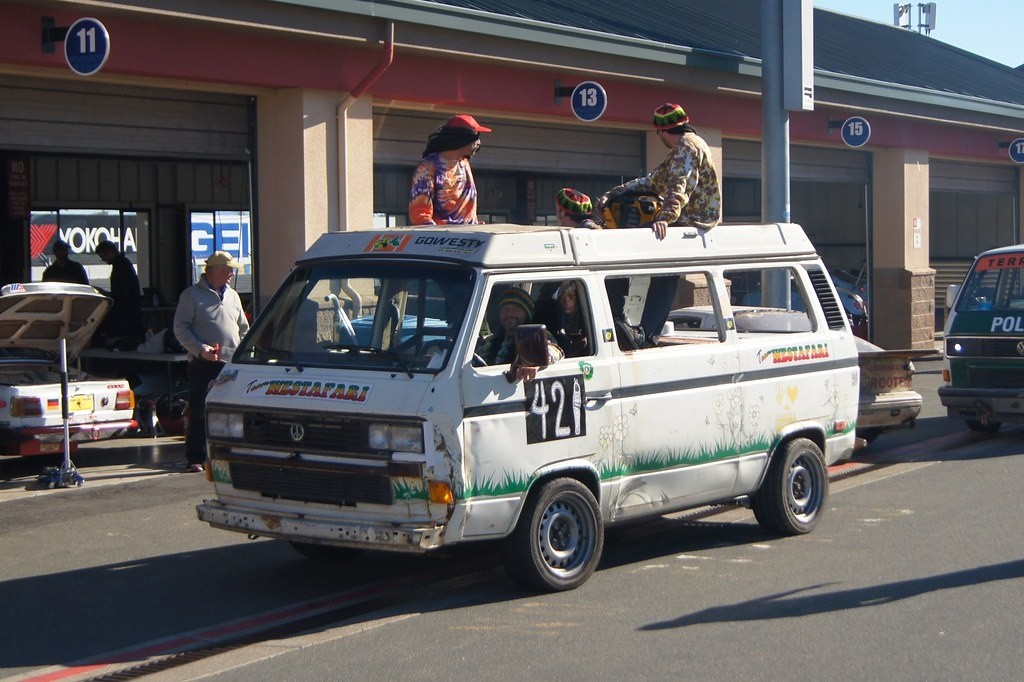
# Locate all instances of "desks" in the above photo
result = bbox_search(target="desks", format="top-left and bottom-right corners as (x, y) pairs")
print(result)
(81, 347), (189, 436)
(140, 305), (178, 329)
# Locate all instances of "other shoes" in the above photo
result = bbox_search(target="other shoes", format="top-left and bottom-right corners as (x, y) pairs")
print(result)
(186, 464), (203, 472)
(613, 316), (646, 351)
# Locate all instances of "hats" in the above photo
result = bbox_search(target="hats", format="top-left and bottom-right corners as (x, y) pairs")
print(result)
(204, 250), (241, 269)
(555, 188), (592, 215)
(500, 287), (535, 321)
(447, 114), (493, 133)
(652, 102), (689, 130)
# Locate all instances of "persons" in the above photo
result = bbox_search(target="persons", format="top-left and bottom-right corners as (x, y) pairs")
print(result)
(596, 101), (721, 339)
(464, 189), (605, 380)
(41, 240), (182, 398)
(174, 251), (254, 473)
(410, 113), (492, 226)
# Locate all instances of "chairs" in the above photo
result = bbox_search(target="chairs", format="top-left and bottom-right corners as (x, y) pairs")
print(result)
(533, 295), (572, 348)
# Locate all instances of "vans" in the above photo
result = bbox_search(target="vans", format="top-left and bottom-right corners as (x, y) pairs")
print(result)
(194, 224), (860, 596)
(937, 243), (1024, 435)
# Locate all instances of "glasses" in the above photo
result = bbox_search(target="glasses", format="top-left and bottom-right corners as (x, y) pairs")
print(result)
(656, 129), (659, 134)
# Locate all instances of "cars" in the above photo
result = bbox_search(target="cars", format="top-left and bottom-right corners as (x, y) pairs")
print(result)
(661, 305), (941, 445)
(0, 280), (138, 453)
